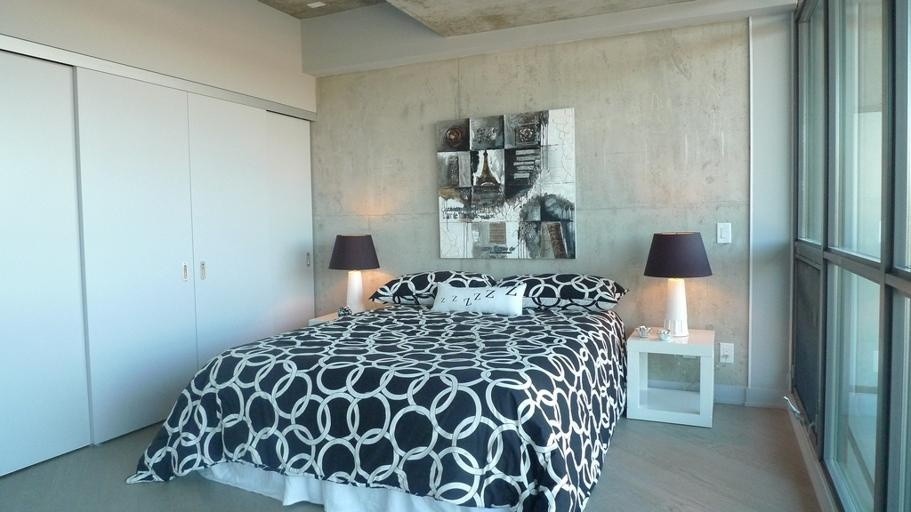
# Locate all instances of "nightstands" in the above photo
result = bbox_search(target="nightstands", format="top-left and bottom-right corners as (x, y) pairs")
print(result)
(309, 312), (339, 326)
(626, 327), (715, 429)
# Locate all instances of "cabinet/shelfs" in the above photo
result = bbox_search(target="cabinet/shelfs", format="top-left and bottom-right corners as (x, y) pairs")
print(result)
(268, 108), (315, 338)
(73, 66), (277, 446)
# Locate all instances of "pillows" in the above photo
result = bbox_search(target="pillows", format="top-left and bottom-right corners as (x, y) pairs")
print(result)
(431, 282), (527, 316)
(494, 273), (630, 312)
(368, 270), (501, 305)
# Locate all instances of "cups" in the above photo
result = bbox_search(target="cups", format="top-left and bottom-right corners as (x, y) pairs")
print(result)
(635, 325), (672, 341)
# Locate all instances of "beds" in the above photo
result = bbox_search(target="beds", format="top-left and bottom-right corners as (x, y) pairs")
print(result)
(125, 307), (626, 512)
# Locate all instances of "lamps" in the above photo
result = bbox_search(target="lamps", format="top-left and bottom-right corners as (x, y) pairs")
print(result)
(328, 234), (380, 314)
(644, 232), (713, 336)
